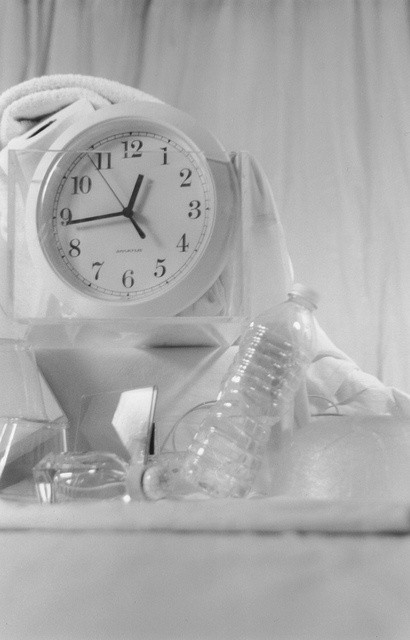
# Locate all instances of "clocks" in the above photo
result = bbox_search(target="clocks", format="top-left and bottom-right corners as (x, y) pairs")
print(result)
(21, 98), (239, 317)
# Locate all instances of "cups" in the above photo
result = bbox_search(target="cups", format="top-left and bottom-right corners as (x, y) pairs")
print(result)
(1, 417), (66, 502)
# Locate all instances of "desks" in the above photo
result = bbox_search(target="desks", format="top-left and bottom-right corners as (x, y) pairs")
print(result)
(0, 487), (410, 638)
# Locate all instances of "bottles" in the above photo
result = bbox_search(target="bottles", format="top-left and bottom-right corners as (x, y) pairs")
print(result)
(179, 282), (319, 497)
